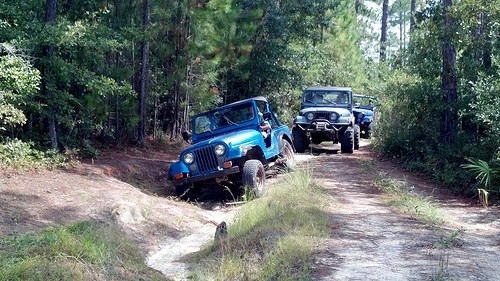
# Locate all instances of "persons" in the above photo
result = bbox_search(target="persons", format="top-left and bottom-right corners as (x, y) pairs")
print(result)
(247, 107), (270, 130)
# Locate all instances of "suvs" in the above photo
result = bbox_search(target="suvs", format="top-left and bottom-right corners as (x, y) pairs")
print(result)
(165, 95), (296, 201)
(292, 86), (360, 153)
(349, 94), (382, 139)
